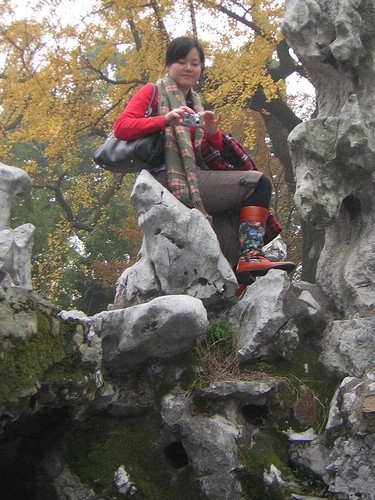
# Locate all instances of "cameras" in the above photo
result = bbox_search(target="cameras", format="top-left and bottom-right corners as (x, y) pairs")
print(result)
(182, 112), (204, 127)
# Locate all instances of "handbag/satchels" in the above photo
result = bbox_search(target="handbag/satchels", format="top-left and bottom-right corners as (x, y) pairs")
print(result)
(94, 83), (163, 173)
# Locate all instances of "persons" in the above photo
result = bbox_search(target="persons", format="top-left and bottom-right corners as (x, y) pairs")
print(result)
(113, 36), (297, 285)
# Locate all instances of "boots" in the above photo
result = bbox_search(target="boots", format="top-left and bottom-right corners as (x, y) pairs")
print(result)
(236, 206), (296, 285)
